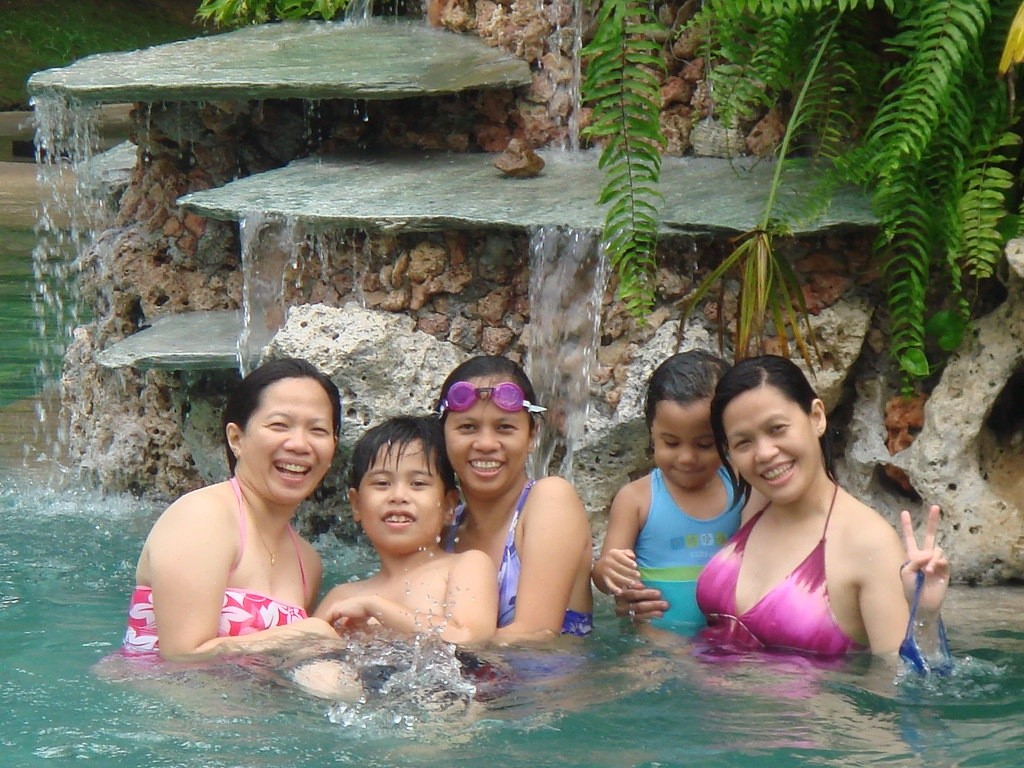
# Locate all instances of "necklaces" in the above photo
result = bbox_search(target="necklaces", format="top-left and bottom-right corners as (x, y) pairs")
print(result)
(242, 499), (282, 565)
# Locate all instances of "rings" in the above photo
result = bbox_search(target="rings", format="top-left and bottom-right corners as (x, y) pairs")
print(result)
(628, 610), (638, 624)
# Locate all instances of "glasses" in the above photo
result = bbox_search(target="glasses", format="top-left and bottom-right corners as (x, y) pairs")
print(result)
(899, 639), (955, 678)
(445, 381), (525, 411)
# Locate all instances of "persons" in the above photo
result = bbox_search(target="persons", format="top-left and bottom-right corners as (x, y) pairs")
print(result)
(432, 355), (595, 703)
(589, 348), (748, 682)
(612, 354), (950, 701)
(311, 415), (500, 697)
(91, 357), (345, 740)
